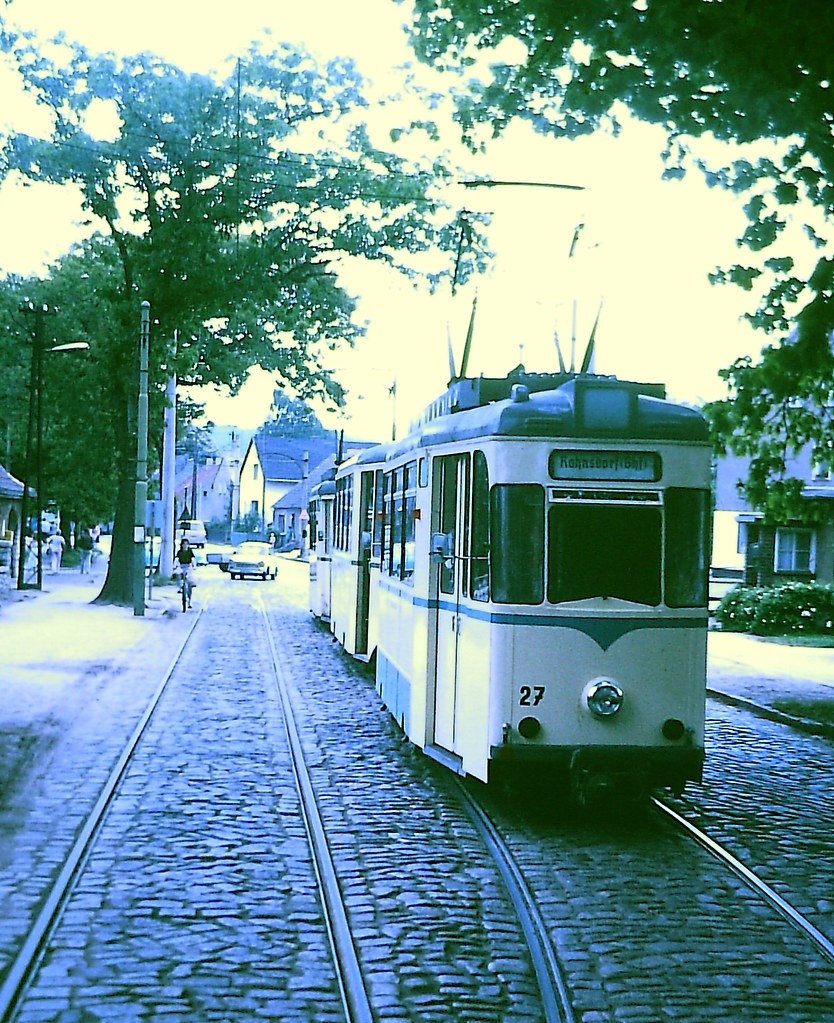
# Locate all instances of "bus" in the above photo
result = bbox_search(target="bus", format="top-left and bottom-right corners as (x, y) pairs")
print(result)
(310, 373), (713, 805)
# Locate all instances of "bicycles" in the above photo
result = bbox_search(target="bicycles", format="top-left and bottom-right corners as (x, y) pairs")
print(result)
(175, 568), (196, 613)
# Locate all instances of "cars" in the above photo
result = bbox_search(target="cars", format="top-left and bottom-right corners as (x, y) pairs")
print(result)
(226, 541), (278, 580)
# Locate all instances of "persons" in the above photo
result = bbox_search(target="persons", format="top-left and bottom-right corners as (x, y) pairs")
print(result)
(171, 537), (197, 609)
(77, 528), (93, 573)
(47, 529), (65, 575)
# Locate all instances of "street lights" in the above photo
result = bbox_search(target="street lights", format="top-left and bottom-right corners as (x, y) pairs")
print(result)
(37, 341), (90, 589)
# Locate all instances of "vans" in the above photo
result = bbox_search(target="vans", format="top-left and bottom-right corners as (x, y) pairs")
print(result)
(175, 520), (207, 549)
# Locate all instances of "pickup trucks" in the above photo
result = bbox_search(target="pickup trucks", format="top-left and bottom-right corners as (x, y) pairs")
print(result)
(206, 553), (235, 572)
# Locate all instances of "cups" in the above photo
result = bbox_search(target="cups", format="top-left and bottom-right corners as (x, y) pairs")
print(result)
(5, 530), (13, 540)
